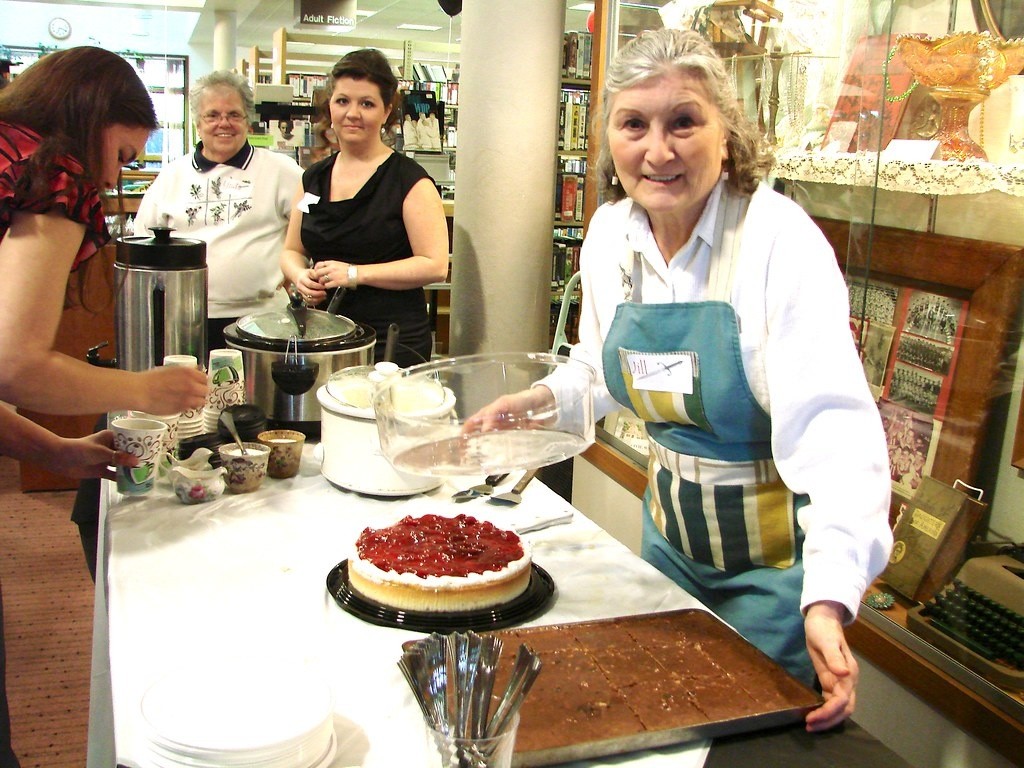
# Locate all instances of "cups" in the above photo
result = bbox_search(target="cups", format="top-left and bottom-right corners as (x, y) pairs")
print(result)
(130, 346), (266, 445)
(421, 693), (520, 768)
(110, 417), (169, 498)
(157, 443), (228, 505)
(218, 443), (271, 492)
(257, 430), (307, 479)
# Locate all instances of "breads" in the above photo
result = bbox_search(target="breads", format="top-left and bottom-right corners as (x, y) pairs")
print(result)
(488, 610), (820, 752)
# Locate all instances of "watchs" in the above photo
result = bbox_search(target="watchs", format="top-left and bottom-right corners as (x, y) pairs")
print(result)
(348, 263), (358, 291)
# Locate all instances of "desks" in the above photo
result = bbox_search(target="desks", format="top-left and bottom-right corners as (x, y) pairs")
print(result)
(86, 446), (920, 768)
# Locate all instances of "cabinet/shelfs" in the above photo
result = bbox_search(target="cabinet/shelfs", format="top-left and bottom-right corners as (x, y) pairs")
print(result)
(235, 28), (591, 359)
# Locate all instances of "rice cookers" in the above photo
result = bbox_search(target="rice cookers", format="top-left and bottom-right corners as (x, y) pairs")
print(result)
(83, 227), (210, 391)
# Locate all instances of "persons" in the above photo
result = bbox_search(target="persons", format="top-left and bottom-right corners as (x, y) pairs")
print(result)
(135, 71), (307, 354)
(279, 48), (449, 370)
(0, 46), (212, 483)
(459, 29), (893, 732)
(273, 120), (304, 146)
(877, 294), (965, 491)
(403, 111), (440, 151)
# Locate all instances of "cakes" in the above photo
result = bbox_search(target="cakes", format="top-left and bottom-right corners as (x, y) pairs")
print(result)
(348, 513), (531, 613)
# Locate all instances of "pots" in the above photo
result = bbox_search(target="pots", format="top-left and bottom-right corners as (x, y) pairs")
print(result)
(219, 292), (379, 442)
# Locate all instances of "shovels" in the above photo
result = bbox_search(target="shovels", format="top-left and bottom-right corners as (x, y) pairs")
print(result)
(490, 468), (540, 504)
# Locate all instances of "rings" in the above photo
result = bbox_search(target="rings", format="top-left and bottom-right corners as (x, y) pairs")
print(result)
(324, 274), (330, 281)
(307, 295), (313, 299)
(112, 451), (116, 465)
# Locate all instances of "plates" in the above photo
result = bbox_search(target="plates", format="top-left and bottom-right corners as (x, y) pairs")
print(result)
(132, 666), (337, 768)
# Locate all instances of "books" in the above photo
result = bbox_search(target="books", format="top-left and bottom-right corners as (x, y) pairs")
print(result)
(391, 123), (456, 199)
(256, 69), (331, 202)
(876, 471), (989, 607)
(547, 27), (595, 356)
(394, 63), (458, 112)
